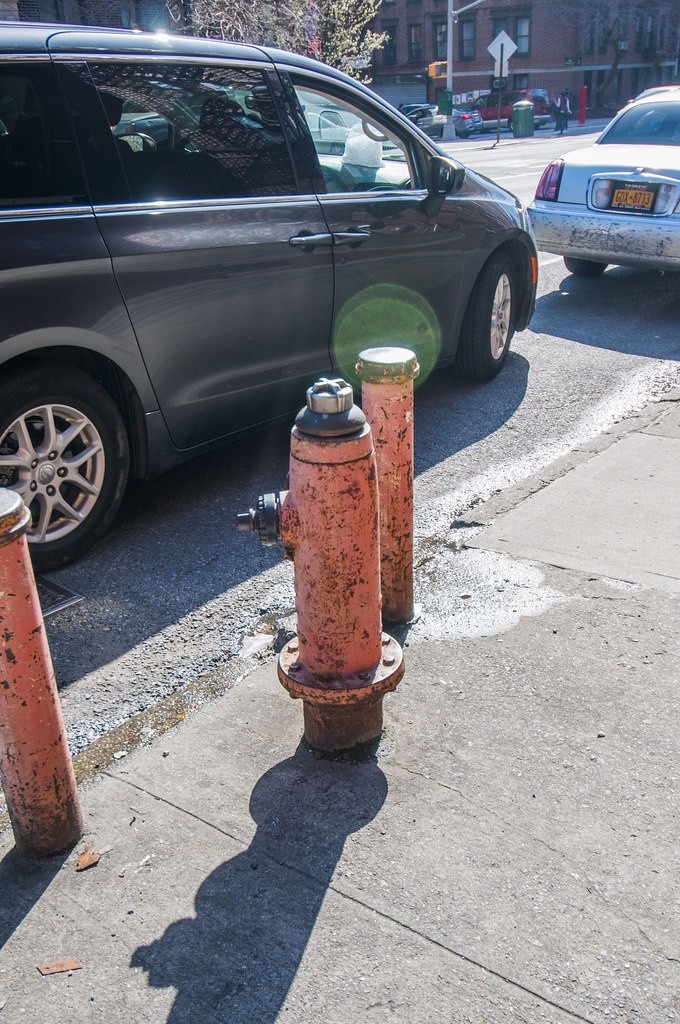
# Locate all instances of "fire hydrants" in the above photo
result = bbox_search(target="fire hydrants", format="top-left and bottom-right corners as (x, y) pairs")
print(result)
(237, 377), (405, 750)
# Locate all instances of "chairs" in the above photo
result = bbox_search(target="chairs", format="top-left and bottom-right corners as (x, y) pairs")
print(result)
(99, 90), (294, 195)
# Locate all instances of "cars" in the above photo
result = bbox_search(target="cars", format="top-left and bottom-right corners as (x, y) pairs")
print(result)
(110, 78), (283, 152)
(399, 104), (483, 139)
(527, 85), (680, 275)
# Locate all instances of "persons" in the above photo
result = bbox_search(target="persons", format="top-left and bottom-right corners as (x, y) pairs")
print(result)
(550, 89), (574, 134)
(409, 111), (424, 118)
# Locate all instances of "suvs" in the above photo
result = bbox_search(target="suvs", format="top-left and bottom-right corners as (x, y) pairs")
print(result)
(0, 22), (539, 576)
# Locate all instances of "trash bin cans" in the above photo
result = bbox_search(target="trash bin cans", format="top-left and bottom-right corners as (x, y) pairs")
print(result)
(512, 100), (534, 138)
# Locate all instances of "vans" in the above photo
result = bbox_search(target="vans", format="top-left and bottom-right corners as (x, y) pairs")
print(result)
(471, 88), (553, 132)
